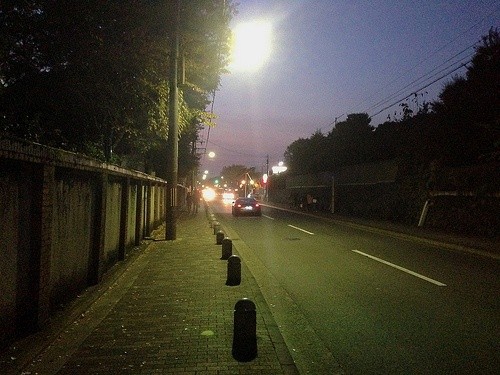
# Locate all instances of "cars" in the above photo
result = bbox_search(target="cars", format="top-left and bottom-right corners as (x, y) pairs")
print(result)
(232, 197), (261, 216)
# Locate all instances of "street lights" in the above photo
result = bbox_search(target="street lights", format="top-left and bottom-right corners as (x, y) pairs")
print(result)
(190, 147), (216, 192)
(164, 17), (267, 243)
(240, 172), (255, 199)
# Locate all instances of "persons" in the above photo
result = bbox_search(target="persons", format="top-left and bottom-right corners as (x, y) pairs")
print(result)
(186, 186), (202, 214)
(291, 190), (324, 214)
